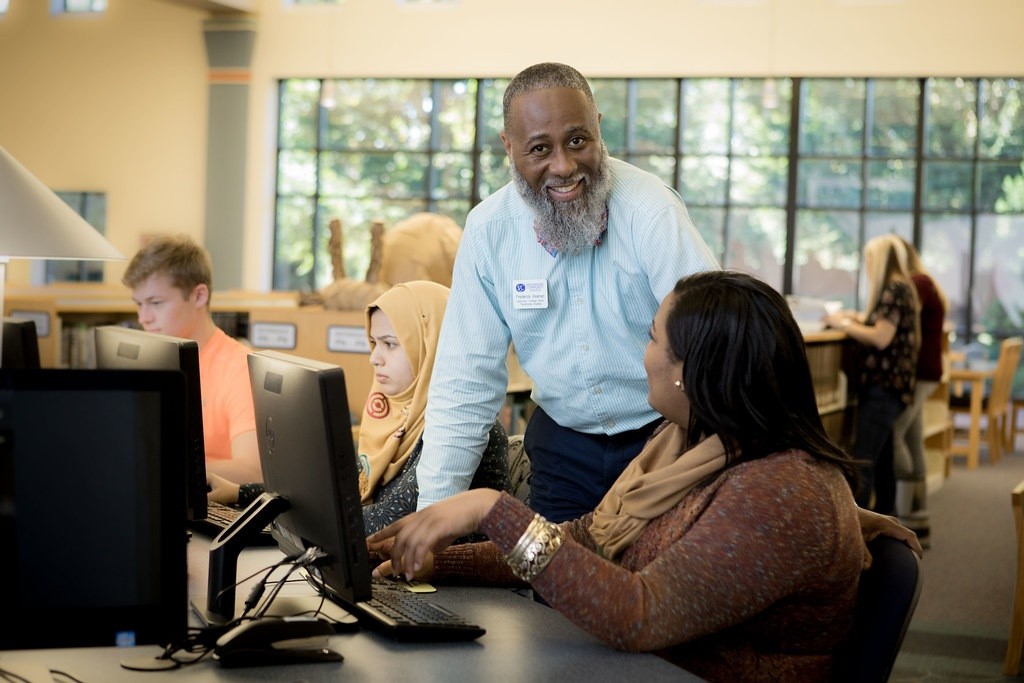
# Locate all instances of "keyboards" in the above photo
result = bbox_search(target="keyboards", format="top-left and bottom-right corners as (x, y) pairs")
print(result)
(305, 574), (487, 643)
(188, 499), (278, 547)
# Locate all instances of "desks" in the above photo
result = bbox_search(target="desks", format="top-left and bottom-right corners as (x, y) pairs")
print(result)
(0, 521), (705, 683)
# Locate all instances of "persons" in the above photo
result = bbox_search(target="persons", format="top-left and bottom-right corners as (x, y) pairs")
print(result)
(821, 235), (919, 520)
(119, 233), (270, 489)
(413, 61), (924, 570)
(205, 280), (513, 555)
(895, 244), (946, 511)
(365, 271), (867, 683)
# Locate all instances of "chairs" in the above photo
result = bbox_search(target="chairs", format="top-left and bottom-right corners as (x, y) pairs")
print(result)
(947, 335), (1024, 465)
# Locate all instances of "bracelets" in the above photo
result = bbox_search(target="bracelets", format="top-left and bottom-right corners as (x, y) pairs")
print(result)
(501, 513), (566, 585)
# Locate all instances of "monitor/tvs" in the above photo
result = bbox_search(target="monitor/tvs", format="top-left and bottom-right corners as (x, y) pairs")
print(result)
(190, 348), (371, 635)
(1, 316), (208, 649)
